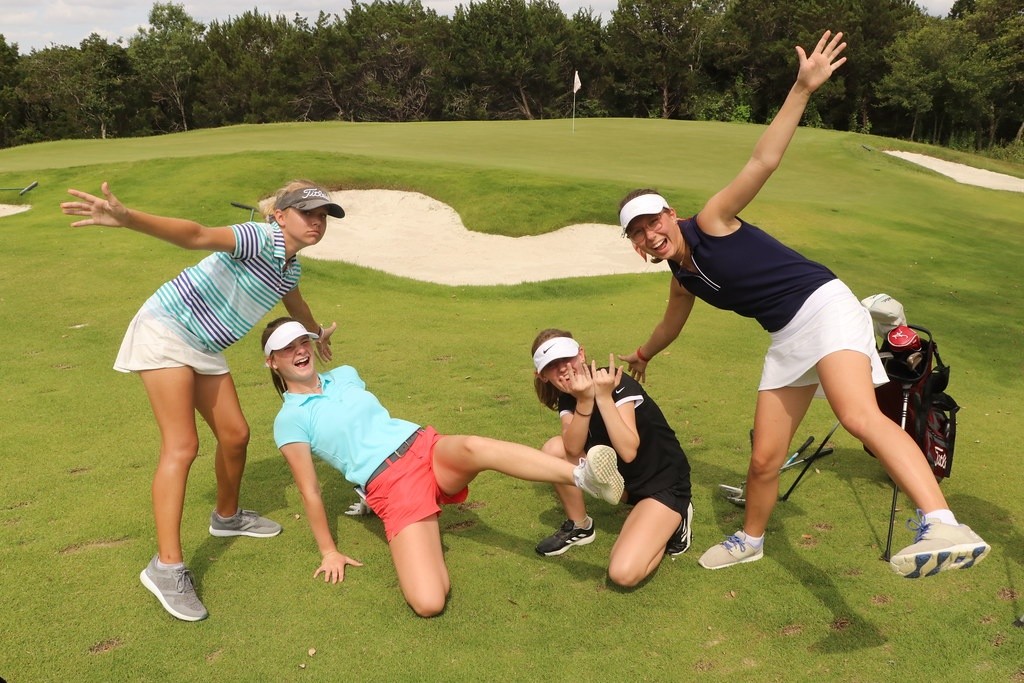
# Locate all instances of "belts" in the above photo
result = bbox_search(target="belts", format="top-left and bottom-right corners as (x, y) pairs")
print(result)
(366, 429), (421, 485)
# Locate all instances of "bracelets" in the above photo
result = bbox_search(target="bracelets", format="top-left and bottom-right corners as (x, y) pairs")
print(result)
(312, 326), (324, 344)
(575, 409), (591, 416)
(637, 346), (649, 362)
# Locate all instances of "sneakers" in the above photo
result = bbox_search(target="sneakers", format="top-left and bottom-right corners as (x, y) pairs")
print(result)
(578, 445), (625, 506)
(535, 515), (596, 556)
(668, 501), (694, 556)
(208, 507), (283, 537)
(890, 508), (992, 578)
(139, 551), (208, 621)
(699, 529), (765, 570)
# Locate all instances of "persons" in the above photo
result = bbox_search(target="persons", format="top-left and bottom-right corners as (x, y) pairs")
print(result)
(531, 328), (693, 588)
(60, 180), (345, 621)
(260, 315), (625, 619)
(617, 30), (991, 578)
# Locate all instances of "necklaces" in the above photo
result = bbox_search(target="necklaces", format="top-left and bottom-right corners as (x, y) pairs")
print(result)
(304, 377), (321, 393)
(677, 247), (687, 274)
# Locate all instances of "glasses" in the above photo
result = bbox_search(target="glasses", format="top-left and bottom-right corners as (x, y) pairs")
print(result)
(626, 209), (667, 244)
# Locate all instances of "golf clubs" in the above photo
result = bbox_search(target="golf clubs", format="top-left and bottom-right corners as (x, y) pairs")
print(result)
(717, 435), (834, 508)
(878, 352), (894, 370)
(907, 351), (925, 372)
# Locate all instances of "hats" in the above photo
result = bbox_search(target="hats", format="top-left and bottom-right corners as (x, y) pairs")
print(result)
(274, 188), (347, 219)
(532, 337), (580, 374)
(263, 320), (320, 359)
(619, 193), (669, 239)
